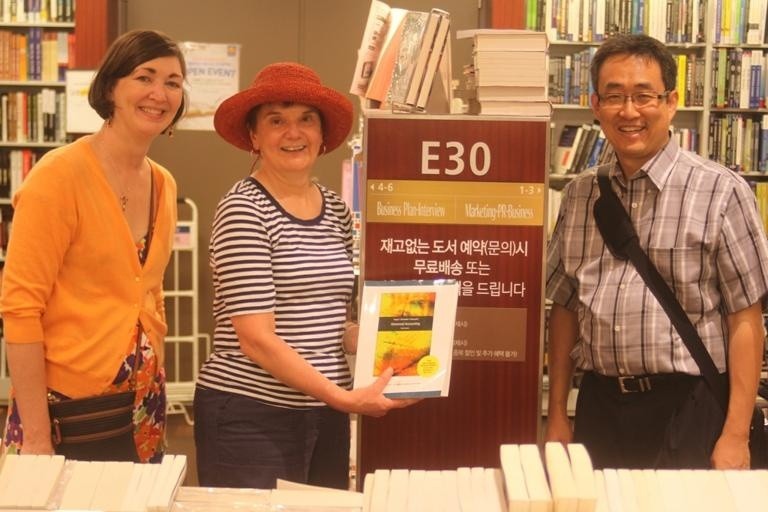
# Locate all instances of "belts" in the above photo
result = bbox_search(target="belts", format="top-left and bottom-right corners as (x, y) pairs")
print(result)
(589, 371), (679, 394)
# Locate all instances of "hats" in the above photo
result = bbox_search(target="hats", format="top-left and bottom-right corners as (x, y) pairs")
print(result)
(212, 63), (354, 155)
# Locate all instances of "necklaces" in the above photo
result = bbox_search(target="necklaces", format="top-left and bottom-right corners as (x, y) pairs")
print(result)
(115, 170), (139, 212)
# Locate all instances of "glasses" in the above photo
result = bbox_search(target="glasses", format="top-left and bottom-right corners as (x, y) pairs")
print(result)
(598, 91), (671, 108)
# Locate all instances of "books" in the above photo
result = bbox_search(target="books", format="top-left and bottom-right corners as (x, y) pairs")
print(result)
(1, 1), (77, 258)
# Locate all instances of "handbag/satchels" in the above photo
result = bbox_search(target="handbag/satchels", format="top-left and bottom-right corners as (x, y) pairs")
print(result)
(653, 371), (767, 468)
(46, 391), (140, 462)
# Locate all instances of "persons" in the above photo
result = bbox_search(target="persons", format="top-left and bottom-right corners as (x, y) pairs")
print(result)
(1, 27), (188, 463)
(191, 62), (425, 490)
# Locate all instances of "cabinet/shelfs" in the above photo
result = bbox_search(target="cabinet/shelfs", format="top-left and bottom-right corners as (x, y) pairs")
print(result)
(529, 0), (768, 182)
(0, 1), (108, 207)
